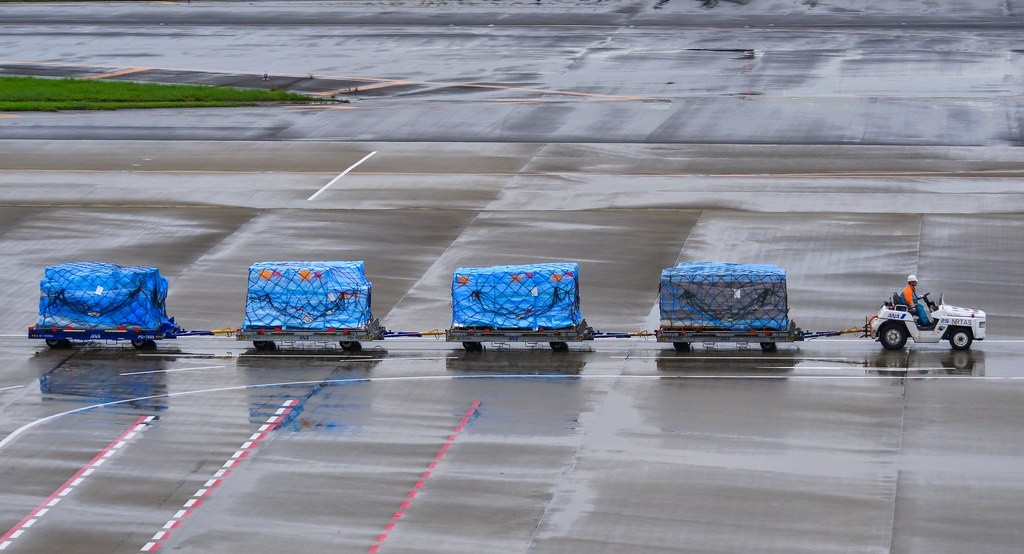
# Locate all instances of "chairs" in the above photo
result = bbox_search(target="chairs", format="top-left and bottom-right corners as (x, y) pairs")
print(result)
(894, 292), (917, 314)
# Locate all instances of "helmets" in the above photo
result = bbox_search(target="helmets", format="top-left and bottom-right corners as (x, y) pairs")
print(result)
(908, 275), (917, 282)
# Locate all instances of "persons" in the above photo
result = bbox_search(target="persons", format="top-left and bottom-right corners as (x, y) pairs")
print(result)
(899, 274), (934, 330)
